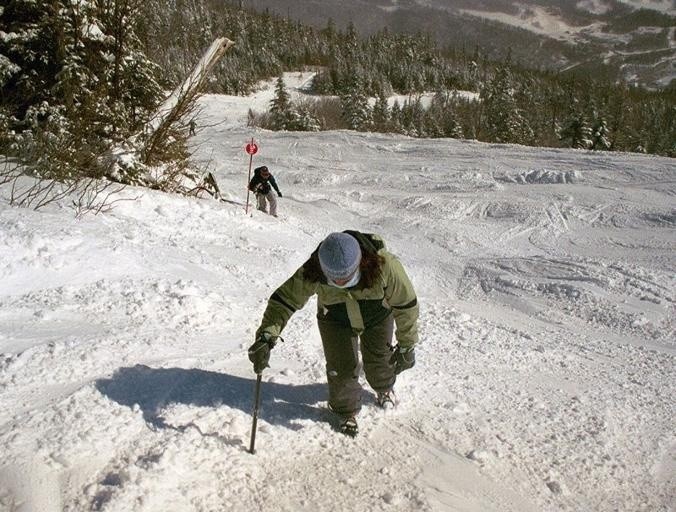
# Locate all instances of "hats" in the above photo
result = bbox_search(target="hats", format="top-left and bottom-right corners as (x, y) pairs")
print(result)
(319, 233), (362, 281)
(260, 166), (269, 175)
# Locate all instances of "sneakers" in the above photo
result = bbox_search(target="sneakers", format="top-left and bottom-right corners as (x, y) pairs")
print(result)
(377, 390), (395, 410)
(336, 414), (359, 438)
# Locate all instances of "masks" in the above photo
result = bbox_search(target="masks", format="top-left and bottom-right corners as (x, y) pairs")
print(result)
(327, 270), (361, 289)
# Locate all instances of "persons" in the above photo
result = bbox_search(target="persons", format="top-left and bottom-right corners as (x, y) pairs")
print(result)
(247, 229), (420, 438)
(248, 165), (283, 219)
(187, 120), (198, 137)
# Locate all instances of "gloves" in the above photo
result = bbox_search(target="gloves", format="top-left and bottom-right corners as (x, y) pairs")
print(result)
(248, 334), (277, 374)
(388, 346), (415, 374)
(278, 191), (281, 197)
